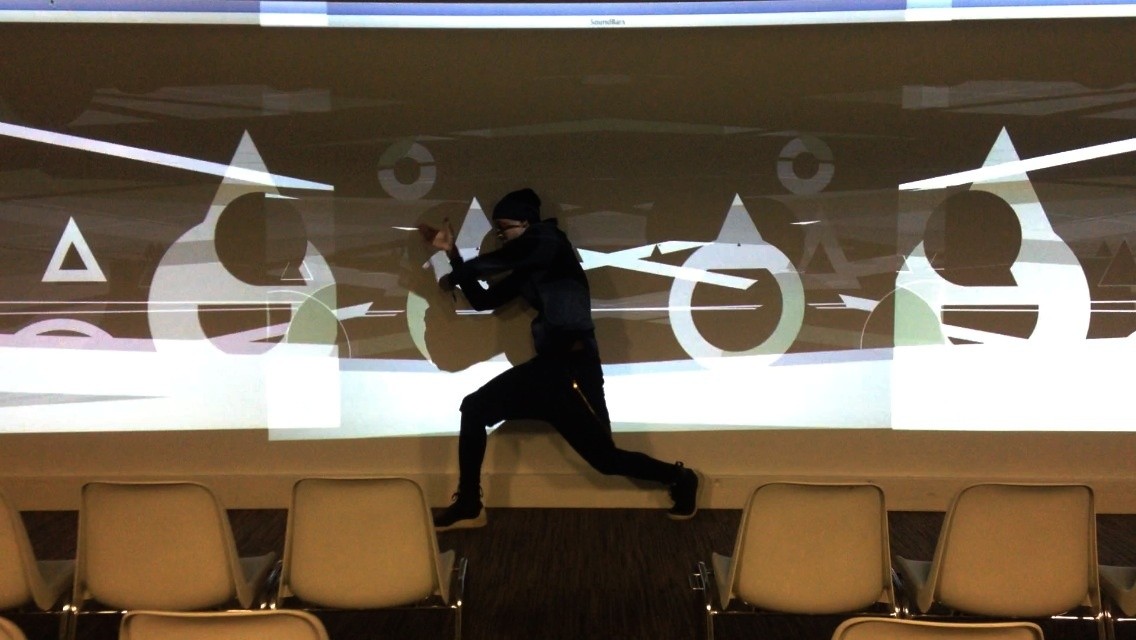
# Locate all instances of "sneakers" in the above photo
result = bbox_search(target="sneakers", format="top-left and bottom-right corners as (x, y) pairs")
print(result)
(667, 461), (701, 521)
(431, 486), (488, 532)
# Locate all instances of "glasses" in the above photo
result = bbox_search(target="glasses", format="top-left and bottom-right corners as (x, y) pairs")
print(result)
(495, 223), (525, 235)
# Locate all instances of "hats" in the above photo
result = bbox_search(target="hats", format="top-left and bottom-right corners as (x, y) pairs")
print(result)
(492, 188), (541, 221)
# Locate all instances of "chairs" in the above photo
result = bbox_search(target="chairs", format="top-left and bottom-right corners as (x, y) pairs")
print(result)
(0, 476), (470, 640)
(694, 481), (1136, 640)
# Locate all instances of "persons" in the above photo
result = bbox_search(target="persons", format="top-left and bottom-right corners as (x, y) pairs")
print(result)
(419, 187), (698, 531)
